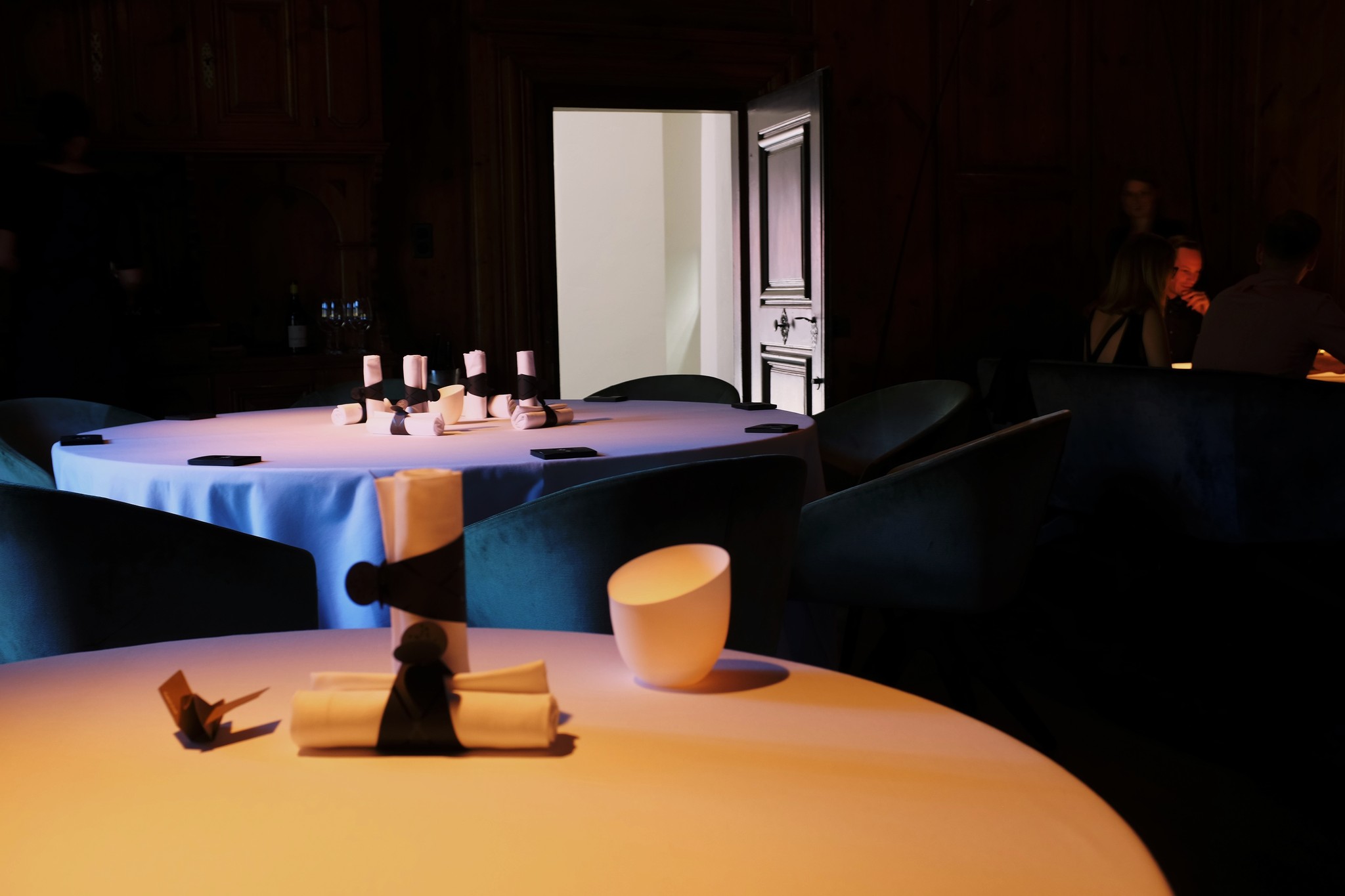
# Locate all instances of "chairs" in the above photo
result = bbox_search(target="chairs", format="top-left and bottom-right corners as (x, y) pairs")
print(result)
(0, 399), (319, 663)
(463, 361), (1344, 896)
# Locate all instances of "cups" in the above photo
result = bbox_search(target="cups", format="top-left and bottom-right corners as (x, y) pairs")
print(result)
(428, 384), (464, 425)
(606, 545), (732, 689)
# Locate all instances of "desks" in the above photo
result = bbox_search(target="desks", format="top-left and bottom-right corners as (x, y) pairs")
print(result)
(1, 620), (1168, 896)
(51, 401), (825, 636)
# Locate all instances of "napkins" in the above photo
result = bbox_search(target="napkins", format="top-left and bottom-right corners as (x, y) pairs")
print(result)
(353, 350), (573, 434)
(291, 618), (562, 752)
(347, 468), (472, 676)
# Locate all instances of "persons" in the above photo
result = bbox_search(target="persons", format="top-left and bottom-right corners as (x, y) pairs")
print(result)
(1085, 160), (1170, 368)
(1157, 243), (1210, 322)
